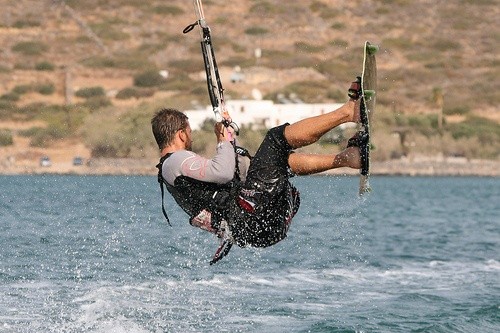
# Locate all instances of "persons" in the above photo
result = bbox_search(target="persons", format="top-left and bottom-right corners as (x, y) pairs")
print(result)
(151, 74), (368, 248)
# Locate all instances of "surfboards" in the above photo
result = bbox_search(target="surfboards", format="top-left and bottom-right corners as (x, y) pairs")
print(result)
(350, 40), (378, 200)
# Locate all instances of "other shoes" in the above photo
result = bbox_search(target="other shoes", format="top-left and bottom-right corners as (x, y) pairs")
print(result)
(347, 130), (364, 169)
(350, 74), (365, 126)
